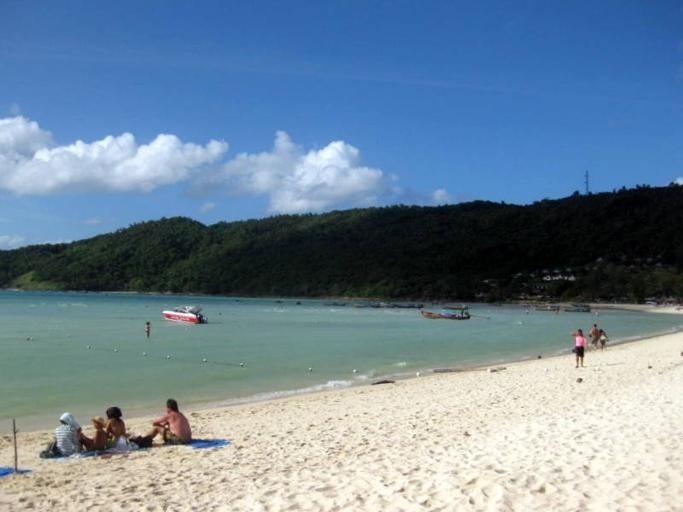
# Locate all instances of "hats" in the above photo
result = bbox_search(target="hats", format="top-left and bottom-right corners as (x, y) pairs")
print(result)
(91, 417), (106, 428)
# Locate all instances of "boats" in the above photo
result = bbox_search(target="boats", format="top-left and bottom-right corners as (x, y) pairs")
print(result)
(535, 305), (590, 312)
(421, 308), (470, 319)
(162, 310), (207, 323)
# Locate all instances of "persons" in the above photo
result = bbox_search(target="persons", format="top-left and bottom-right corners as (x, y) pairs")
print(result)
(76, 415), (107, 451)
(144, 322), (152, 339)
(53, 412), (81, 455)
(588, 323), (599, 347)
(137, 396), (191, 445)
(103, 406), (128, 440)
(598, 329), (610, 350)
(568, 329), (585, 368)
